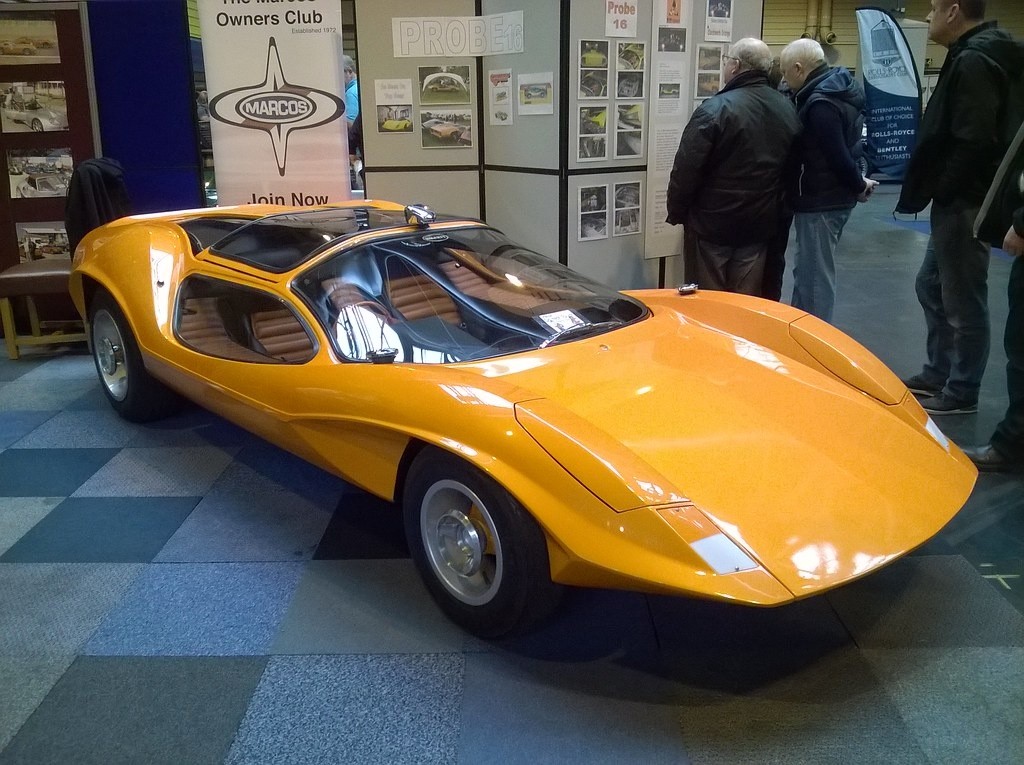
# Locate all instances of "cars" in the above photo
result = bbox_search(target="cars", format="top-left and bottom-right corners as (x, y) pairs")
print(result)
(17, 236), (70, 262)
(524, 86), (546, 100)
(7, 160), (73, 198)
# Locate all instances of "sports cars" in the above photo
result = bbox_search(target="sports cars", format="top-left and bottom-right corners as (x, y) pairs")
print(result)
(423, 114), (471, 145)
(68, 199), (981, 642)
(5, 95), (69, 132)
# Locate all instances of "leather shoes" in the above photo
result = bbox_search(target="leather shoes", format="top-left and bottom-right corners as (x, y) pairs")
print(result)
(959, 445), (1024, 472)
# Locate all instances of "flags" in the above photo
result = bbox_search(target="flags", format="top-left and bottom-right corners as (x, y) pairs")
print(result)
(856, 10), (921, 175)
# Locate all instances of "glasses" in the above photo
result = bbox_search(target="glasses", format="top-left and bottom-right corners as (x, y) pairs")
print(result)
(722, 53), (740, 68)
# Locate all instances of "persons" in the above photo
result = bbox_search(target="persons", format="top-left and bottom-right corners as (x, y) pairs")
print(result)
(664, 39), (880, 324)
(896, 0), (1023, 416)
(342, 55), (364, 191)
(956, 129), (1024, 472)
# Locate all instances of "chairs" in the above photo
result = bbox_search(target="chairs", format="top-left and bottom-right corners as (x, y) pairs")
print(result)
(0, 156), (129, 360)
(182, 256), (548, 366)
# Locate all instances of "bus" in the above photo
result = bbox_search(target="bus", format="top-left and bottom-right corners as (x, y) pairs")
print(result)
(34, 80), (66, 99)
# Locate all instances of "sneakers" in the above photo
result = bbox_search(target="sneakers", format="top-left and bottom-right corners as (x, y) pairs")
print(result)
(903, 375), (941, 396)
(919, 391), (979, 414)
